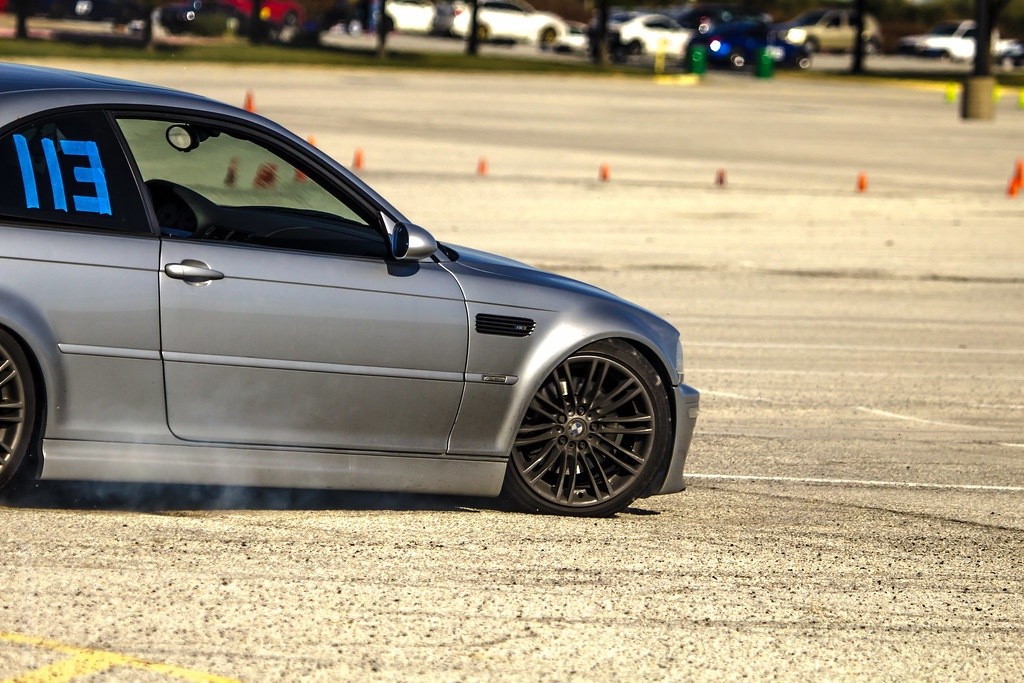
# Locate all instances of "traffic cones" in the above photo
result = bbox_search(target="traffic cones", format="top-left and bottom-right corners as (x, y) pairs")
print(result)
(474, 157), (489, 176)
(1004, 158), (1023, 201)
(854, 169), (870, 195)
(223, 156), (241, 186)
(714, 164), (727, 187)
(242, 89), (257, 112)
(598, 163), (611, 182)
(350, 148), (365, 172)
(294, 135), (316, 183)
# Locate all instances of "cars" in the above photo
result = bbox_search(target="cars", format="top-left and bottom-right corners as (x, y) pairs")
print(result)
(684, 24), (812, 76)
(225, 0), (395, 38)
(347, 1), (450, 36)
(2, 56), (703, 520)
(584, 13), (694, 63)
(657, 0), (785, 33)
(447, 0), (570, 48)
(549, 18), (589, 55)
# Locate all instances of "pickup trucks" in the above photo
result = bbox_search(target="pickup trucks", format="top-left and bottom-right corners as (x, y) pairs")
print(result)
(900, 18), (1022, 72)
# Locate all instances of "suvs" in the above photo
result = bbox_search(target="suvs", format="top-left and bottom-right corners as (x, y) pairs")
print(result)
(780, 6), (884, 56)
